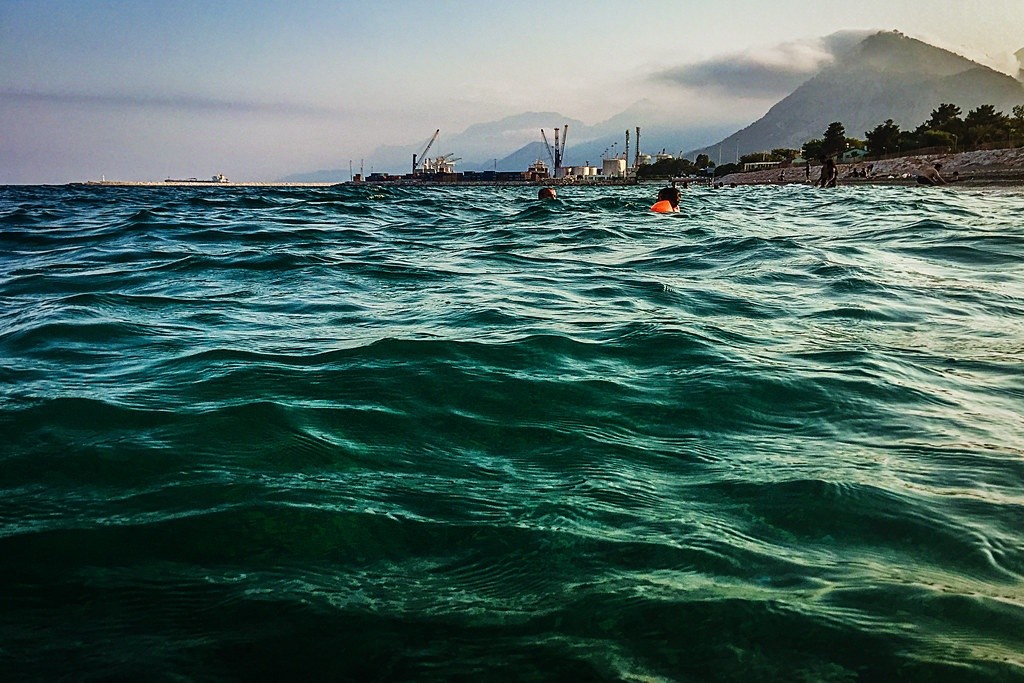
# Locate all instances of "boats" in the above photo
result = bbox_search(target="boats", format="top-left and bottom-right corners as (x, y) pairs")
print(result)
(165, 172), (229, 183)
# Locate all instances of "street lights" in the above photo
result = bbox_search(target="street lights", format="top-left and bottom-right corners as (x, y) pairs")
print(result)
(883, 147), (887, 160)
(952, 134), (957, 154)
(896, 146), (900, 157)
(915, 141), (919, 156)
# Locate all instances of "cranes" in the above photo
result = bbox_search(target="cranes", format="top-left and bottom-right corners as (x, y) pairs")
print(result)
(541, 124), (568, 178)
(413, 129), (462, 173)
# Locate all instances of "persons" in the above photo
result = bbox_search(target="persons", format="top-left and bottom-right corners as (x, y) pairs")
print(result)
(652, 188), (681, 214)
(538, 188), (556, 199)
(825, 159), (839, 188)
(917, 164), (946, 186)
(803, 161), (812, 179)
(781, 170), (785, 181)
(847, 163), (874, 177)
(814, 155), (828, 186)
(951, 171), (958, 181)
